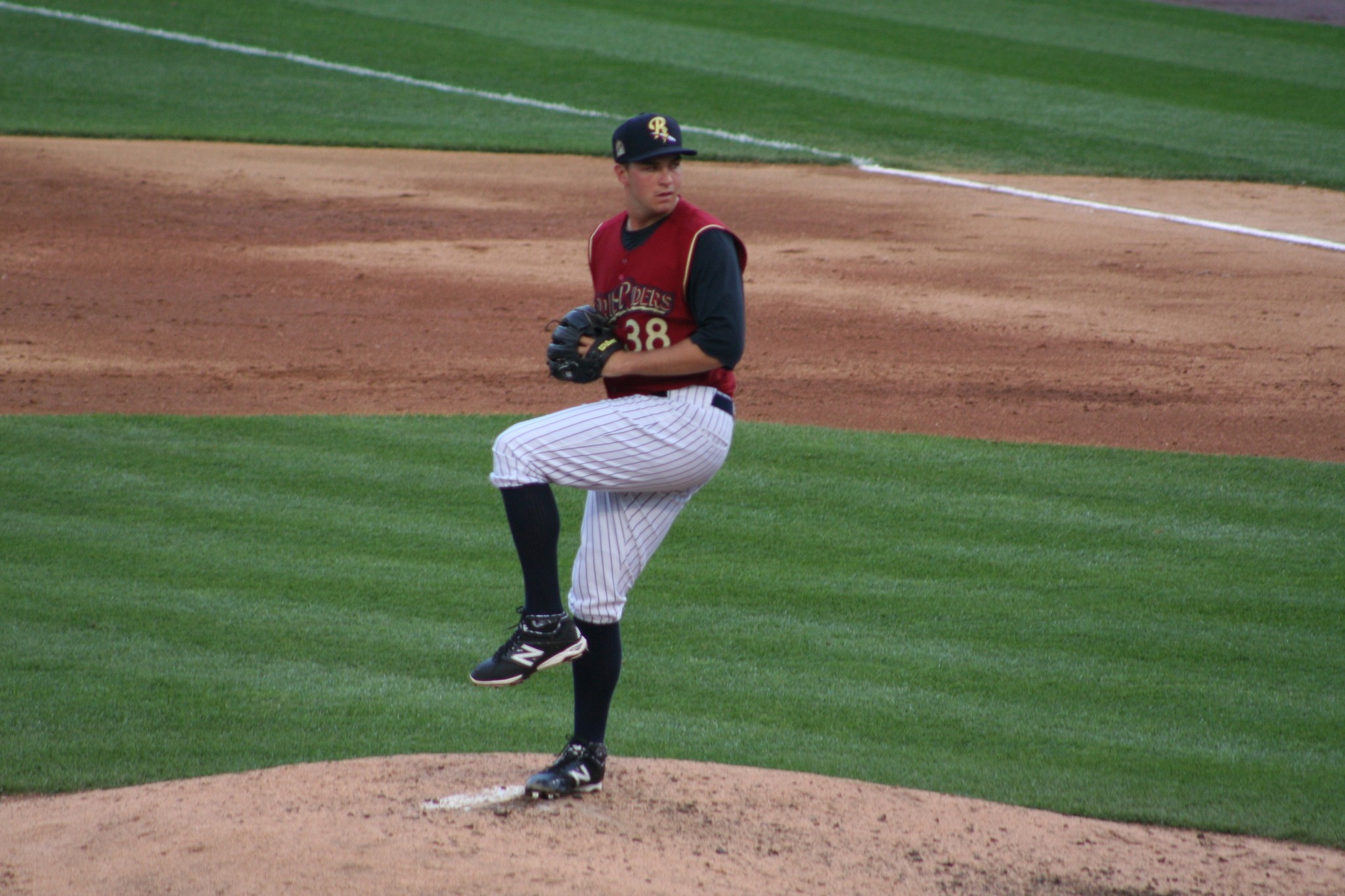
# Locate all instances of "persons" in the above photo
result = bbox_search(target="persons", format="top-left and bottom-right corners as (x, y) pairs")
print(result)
(469, 114), (748, 799)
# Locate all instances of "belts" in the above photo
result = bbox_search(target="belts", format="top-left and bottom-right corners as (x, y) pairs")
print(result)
(651, 390), (734, 417)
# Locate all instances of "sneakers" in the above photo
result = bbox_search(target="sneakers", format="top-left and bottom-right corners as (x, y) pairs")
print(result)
(470, 607), (588, 689)
(524, 736), (607, 799)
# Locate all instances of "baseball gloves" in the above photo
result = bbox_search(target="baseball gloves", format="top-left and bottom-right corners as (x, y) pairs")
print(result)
(549, 305), (620, 386)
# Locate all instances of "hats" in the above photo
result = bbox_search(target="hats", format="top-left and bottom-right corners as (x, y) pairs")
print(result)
(612, 111), (697, 163)
